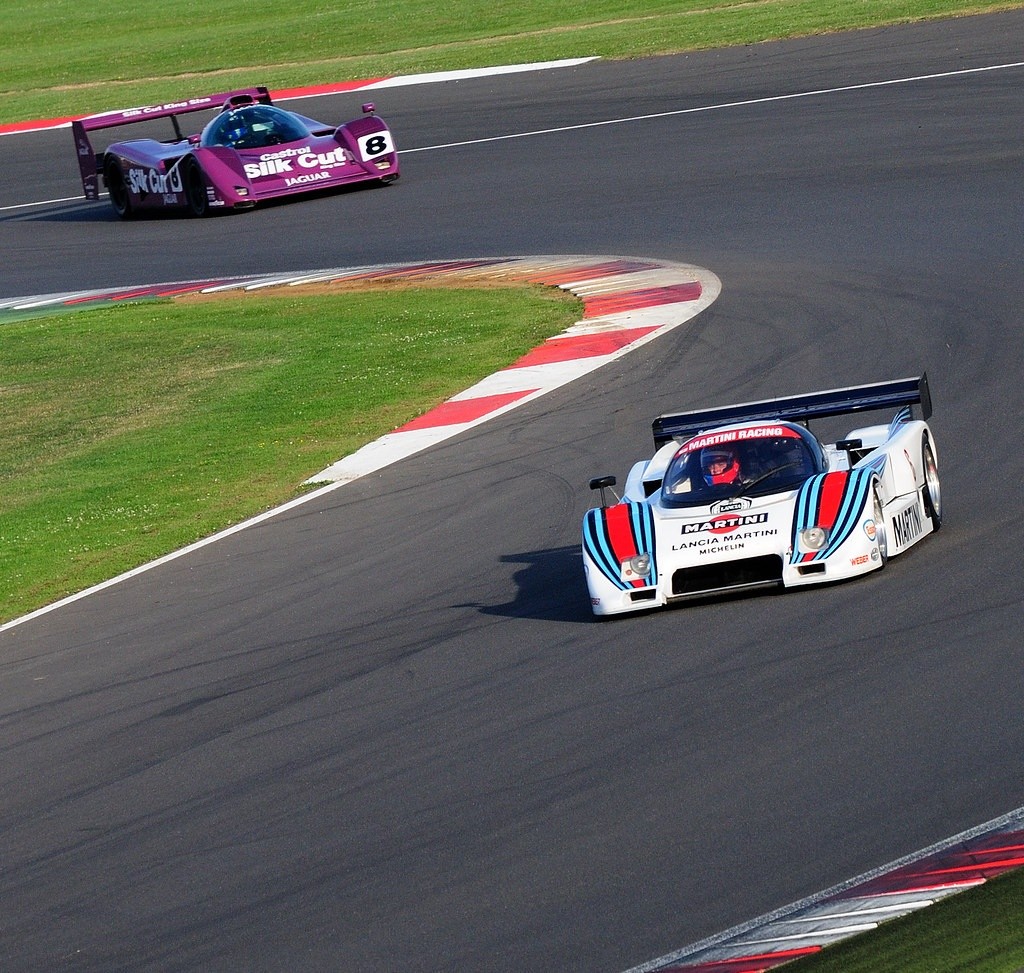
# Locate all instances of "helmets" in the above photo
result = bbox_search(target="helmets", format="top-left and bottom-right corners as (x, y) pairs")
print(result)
(700, 442), (740, 486)
(218, 112), (248, 141)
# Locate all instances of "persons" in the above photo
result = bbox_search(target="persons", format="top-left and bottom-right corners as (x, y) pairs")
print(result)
(698, 443), (755, 491)
(223, 114), (257, 147)
